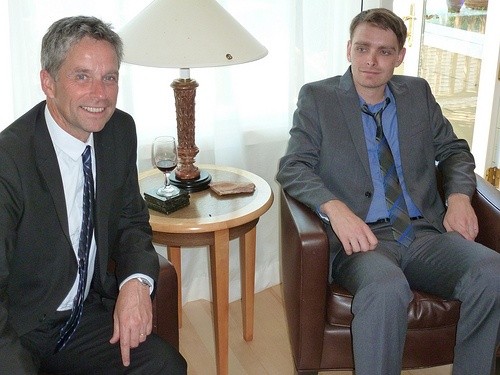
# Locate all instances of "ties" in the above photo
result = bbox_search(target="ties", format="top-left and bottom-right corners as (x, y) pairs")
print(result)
(360, 98), (417, 247)
(53, 145), (94, 354)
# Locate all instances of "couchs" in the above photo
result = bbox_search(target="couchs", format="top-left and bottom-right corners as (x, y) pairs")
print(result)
(281, 174), (500, 375)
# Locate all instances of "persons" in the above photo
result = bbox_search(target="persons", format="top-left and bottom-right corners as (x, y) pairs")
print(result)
(0, 15), (190, 375)
(274, 7), (500, 375)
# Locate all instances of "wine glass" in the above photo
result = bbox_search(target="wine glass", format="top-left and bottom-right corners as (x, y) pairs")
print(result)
(153, 136), (180, 197)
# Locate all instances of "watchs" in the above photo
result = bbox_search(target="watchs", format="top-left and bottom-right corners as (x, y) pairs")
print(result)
(135, 277), (152, 288)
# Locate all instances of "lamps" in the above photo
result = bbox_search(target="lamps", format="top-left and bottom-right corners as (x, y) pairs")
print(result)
(116, 0), (268, 191)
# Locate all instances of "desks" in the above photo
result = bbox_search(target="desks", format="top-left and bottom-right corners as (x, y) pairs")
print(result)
(138, 163), (274, 375)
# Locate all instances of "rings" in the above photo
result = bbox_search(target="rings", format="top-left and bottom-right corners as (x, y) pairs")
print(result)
(139, 333), (146, 335)
